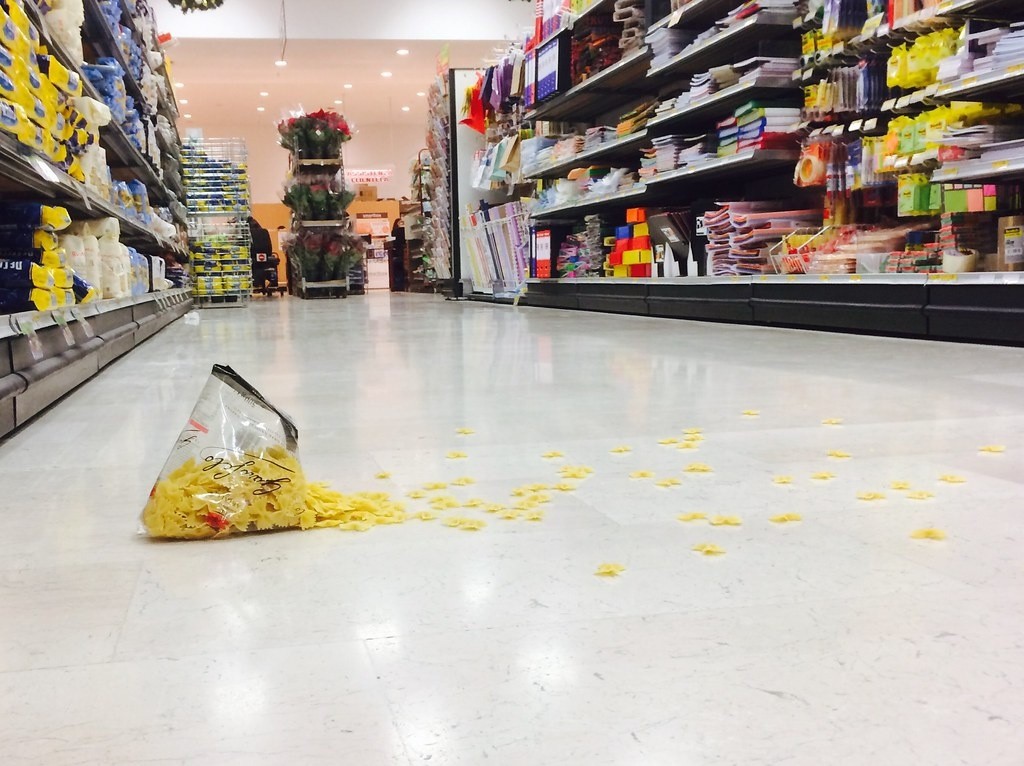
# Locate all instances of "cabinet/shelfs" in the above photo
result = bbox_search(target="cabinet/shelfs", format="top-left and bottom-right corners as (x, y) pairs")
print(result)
(180, 138), (252, 309)
(289, 136), (350, 298)
(0, 1), (196, 442)
(523, 0), (1024, 347)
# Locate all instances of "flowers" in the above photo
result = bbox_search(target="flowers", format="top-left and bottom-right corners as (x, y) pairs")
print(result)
(278, 171), (355, 220)
(277, 104), (355, 158)
(279, 227), (366, 280)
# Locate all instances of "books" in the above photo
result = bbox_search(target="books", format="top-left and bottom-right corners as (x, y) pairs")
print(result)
(636, 0), (800, 180)
(937, 20), (1024, 160)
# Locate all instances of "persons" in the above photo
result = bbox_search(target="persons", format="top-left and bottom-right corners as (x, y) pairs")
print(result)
(391, 218), (407, 285)
(246, 216), (273, 286)
